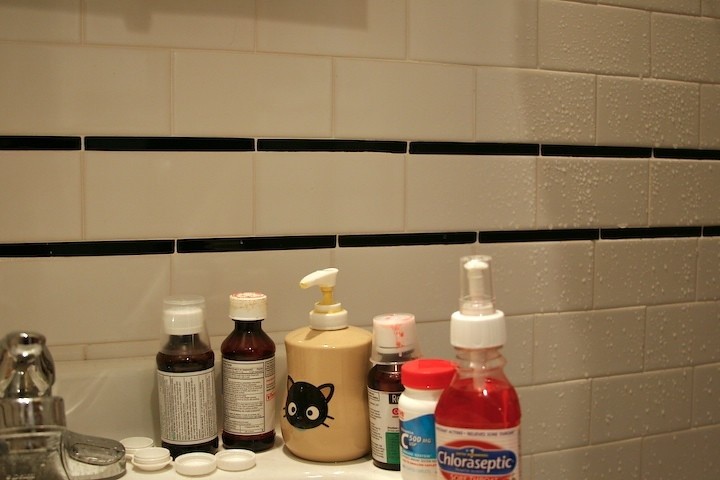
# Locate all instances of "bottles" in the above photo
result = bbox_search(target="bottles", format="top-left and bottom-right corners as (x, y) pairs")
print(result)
(366, 312), (417, 472)
(156, 294), (219, 459)
(397, 358), (459, 480)
(220, 291), (280, 452)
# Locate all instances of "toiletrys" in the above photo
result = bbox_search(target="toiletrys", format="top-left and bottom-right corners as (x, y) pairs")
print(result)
(281, 267), (373, 463)
(434, 253), (522, 480)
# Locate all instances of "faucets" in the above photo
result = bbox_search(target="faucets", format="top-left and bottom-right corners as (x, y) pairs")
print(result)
(0, 330), (127, 480)
